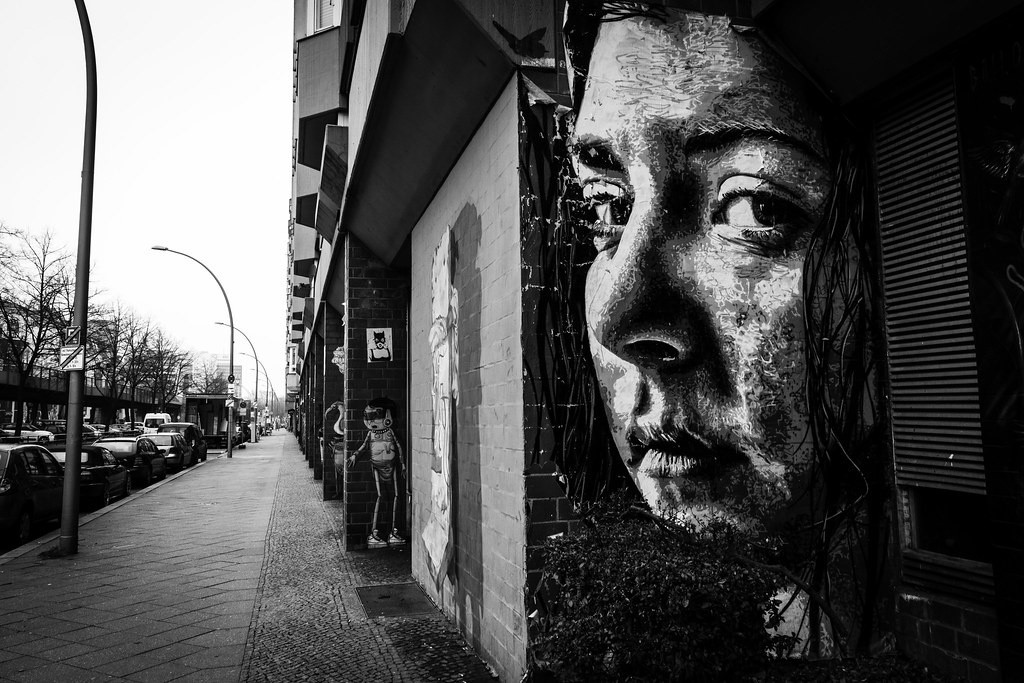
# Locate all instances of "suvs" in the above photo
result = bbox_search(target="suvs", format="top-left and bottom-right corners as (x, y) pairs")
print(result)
(157, 422), (208, 464)
(0, 441), (66, 547)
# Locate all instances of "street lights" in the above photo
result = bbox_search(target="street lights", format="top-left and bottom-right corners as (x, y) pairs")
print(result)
(240, 352), (269, 436)
(215, 321), (259, 442)
(251, 369), (274, 428)
(150, 245), (235, 458)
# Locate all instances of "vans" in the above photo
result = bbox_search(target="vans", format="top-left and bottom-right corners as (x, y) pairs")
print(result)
(143, 412), (172, 434)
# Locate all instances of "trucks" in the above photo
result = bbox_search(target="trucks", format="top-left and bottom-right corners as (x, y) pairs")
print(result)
(182, 393), (247, 449)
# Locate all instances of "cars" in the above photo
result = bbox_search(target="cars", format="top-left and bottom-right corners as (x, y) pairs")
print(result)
(140, 432), (193, 469)
(90, 434), (167, 488)
(27, 444), (132, 508)
(0, 420), (144, 445)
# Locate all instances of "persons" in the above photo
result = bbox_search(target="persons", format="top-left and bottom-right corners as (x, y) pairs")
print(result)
(183, 423), (200, 465)
